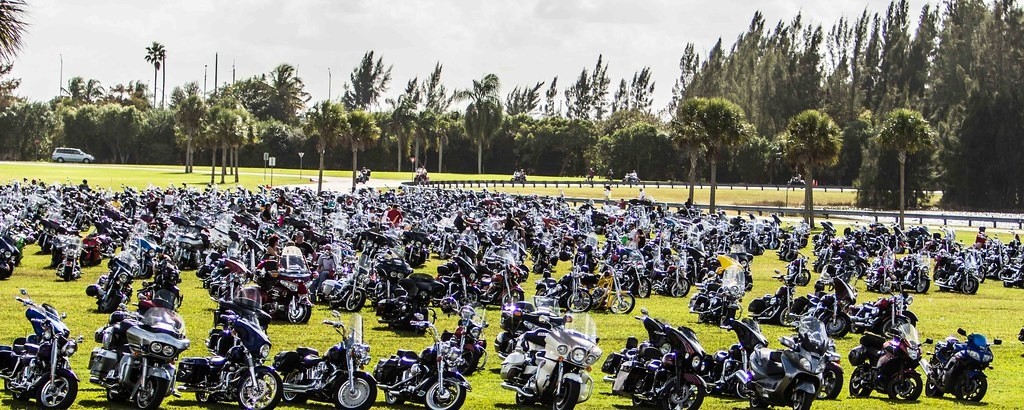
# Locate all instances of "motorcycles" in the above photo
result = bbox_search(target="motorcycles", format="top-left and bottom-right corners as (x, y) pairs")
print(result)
(0, 169), (1024, 410)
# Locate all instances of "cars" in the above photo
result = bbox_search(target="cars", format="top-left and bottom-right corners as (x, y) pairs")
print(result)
(52, 147), (95, 164)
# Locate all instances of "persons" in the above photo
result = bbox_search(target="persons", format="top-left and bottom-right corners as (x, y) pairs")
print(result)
(417, 166), (427, 181)
(604, 186), (611, 204)
(588, 168), (594, 180)
(500, 212), (525, 237)
(514, 168), (525, 180)
(606, 169), (613, 180)
(454, 211), (474, 233)
(228, 197), (319, 263)
(636, 188), (645, 201)
(619, 199), (626, 209)
(368, 204), (405, 229)
(634, 229), (645, 248)
(361, 167), (367, 182)
